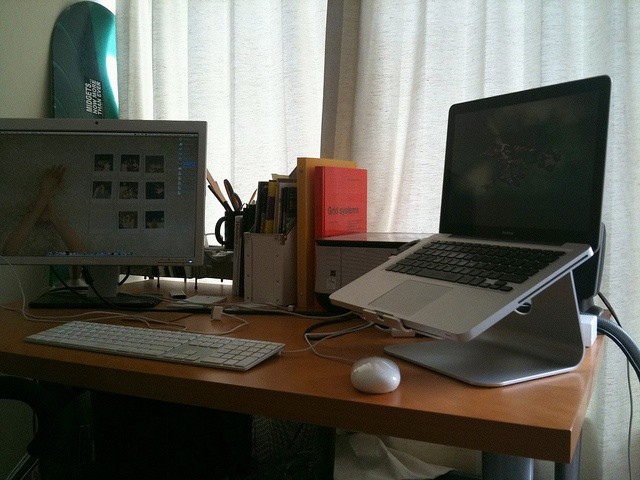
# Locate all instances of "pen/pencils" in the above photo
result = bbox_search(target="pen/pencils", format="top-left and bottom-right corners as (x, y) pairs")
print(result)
(247, 187), (260, 206)
(207, 168), (234, 216)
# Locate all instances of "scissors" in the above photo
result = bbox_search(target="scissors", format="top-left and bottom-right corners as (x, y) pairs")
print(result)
(224, 179), (242, 212)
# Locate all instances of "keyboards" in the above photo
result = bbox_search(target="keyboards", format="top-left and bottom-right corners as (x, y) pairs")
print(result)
(22, 318), (286, 372)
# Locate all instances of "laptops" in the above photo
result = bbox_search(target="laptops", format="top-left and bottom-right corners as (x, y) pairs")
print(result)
(328, 74), (612, 344)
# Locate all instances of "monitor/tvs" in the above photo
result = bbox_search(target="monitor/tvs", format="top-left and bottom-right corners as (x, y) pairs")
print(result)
(0, 117), (208, 311)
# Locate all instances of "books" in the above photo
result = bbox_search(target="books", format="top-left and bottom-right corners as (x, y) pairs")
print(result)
(232, 155), (367, 313)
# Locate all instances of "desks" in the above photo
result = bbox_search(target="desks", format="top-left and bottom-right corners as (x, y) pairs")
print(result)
(0, 280), (613, 480)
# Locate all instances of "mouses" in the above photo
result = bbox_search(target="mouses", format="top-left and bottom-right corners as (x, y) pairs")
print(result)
(350, 356), (402, 394)
